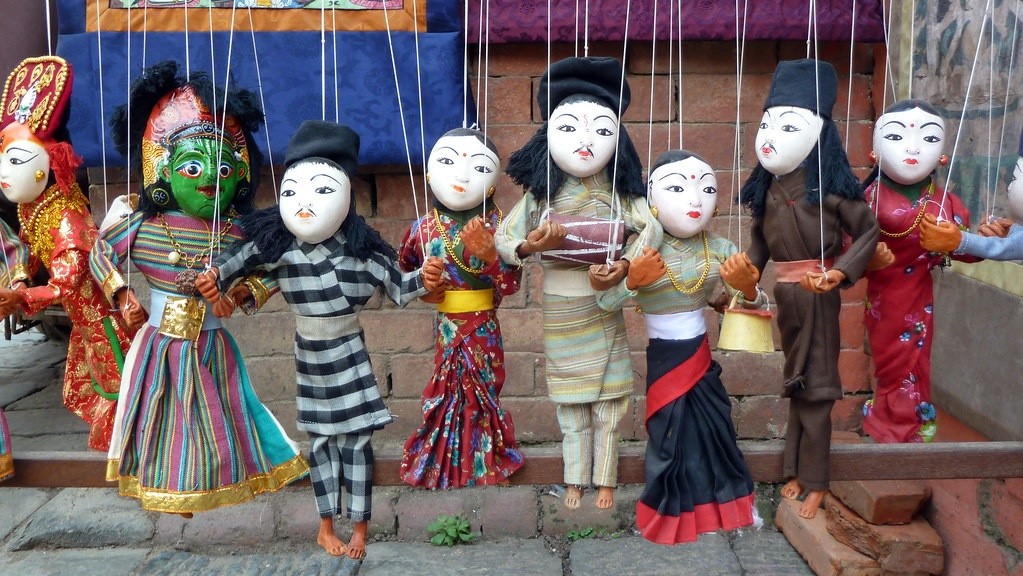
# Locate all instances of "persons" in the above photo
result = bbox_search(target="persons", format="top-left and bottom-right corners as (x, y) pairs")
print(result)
(713, 60), (879, 520)
(596, 150), (763, 544)
(919, 125), (1023, 261)
(89, 84), (311, 518)
(0, 56), (150, 452)
(846, 100), (1004, 444)
(0, 219), (33, 483)
(194, 122), (445, 558)
(493, 56), (664, 508)
(398, 126), (525, 489)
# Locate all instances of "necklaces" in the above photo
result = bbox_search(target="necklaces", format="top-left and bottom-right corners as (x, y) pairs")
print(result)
(17, 190), (63, 249)
(433, 200), (503, 273)
(870, 182), (933, 237)
(157, 213), (232, 295)
(665, 230), (710, 294)
